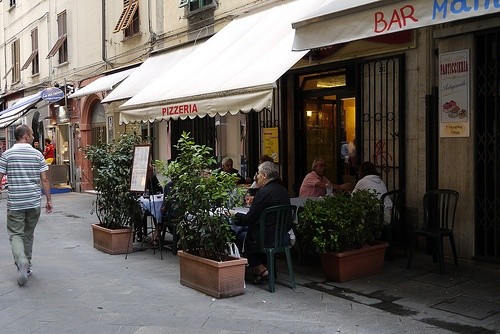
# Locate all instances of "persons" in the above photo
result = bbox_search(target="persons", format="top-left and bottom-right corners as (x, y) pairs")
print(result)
(224, 161), (293, 284)
(300, 158), (351, 199)
(0, 125), (54, 289)
(130, 154), (280, 247)
(347, 162), (400, 235)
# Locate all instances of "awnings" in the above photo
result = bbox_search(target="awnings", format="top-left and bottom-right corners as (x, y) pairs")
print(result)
(67, 65), (137, 102)
(0, 84), (63, 132)
(290, 0), (498, 57)
(100, 36), (211, 107)
(117, 0), (345, 126)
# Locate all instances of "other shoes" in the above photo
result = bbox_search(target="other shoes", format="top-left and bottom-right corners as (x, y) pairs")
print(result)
(17, 257), (28, 286)
(26, 269), (32, 276)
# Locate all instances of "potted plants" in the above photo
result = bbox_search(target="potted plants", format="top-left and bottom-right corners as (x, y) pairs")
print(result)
(151, 130), (250, 299)
(297, 188), (389, 282)
(78, 128), (156, 255)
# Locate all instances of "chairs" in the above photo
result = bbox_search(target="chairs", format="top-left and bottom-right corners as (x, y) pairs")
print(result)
(376, 189), (409, 261)
(257, 205), (296, 293)
(125, 195), (179, 260)
(406, 190), (459, 275)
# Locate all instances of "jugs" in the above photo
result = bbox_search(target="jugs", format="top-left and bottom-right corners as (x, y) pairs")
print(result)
(143, 189), (149, 199)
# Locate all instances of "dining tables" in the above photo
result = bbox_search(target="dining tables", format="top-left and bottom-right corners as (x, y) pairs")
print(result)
(185, 196), (327, 257)
(125, 187), (249, 246)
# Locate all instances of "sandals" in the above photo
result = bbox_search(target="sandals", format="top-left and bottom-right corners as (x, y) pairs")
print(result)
(253, 268), (269, 285)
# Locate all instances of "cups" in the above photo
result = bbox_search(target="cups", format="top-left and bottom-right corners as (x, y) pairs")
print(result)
(157, 192), (161, 198)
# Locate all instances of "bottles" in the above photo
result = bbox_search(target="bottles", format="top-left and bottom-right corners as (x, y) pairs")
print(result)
(326, 180), (332, 194)
